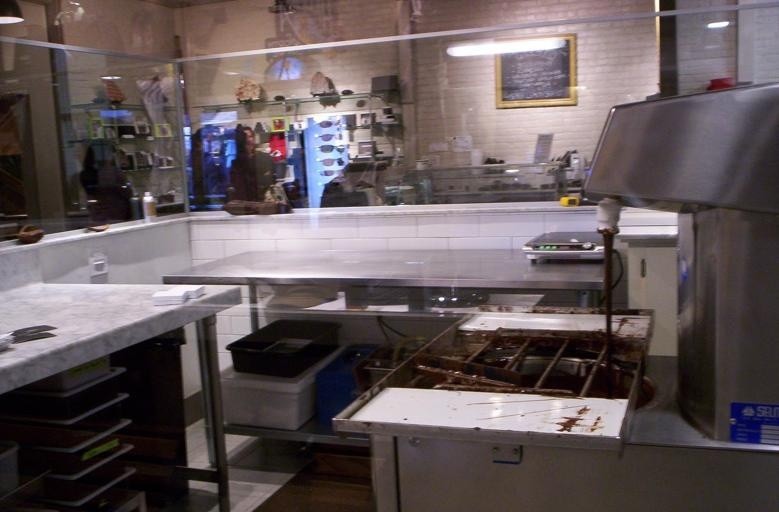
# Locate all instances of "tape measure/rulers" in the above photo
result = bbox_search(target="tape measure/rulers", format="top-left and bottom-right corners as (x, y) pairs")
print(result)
(559, 196), (579, 207)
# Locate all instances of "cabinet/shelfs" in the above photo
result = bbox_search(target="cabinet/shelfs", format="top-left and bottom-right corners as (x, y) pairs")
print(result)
(431, 162), (590, 197)
(191, 91), (403, 161)
(67, 102), (184, 218)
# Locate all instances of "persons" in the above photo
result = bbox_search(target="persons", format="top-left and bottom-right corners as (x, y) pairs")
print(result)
(231, 126), (277, 201)
(77, 136), (135, 220)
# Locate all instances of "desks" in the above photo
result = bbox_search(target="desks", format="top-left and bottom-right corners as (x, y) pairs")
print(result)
(163, 250), (629, 473)
(0, 284), (240, 512)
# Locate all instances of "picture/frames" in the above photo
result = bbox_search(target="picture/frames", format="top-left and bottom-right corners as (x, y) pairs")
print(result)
(495, 33), (578, 109)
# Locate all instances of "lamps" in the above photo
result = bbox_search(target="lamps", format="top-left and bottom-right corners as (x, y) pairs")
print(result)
(0, 0), (25, 24)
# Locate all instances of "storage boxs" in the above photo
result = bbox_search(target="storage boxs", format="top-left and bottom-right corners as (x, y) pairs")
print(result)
(221, 318), (380, 432)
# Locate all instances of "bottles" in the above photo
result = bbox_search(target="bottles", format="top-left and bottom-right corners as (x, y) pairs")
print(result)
(142, 191), (157, 220)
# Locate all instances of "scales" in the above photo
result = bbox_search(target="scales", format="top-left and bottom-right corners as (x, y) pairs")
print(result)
(522, 231), (605, 264)
